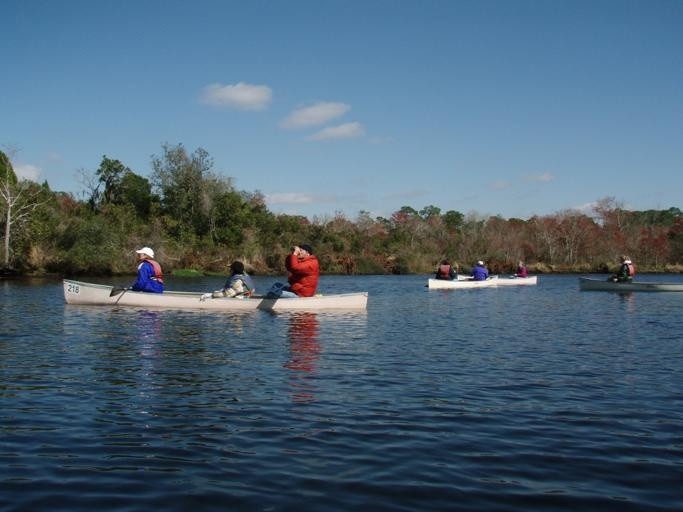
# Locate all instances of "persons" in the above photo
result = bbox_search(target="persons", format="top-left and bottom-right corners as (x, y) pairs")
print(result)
(509, 261), (528, 279)
(200, 261), (255, 301)
(265, 244), (320, 299)
(607, 256), (635, 284)
(468, 260), (489, 281)
(131, 247), (164, 294)
(435, 260), (455, 280)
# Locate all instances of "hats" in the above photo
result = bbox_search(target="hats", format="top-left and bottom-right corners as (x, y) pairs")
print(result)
(227, 261), (244, 274)
(136, 246), (154, 259)
(298, 243), (312, 255)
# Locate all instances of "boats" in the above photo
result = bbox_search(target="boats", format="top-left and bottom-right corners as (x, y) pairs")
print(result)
(59, 302), (370, 340)
(577, 276), (683, 293)
(61, 279), (369, 314)
(427, 274), (538, 291)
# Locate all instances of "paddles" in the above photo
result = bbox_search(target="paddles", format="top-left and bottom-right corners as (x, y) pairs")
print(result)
(110, 284), (133, 296)
(269, 282), (290, 296)
